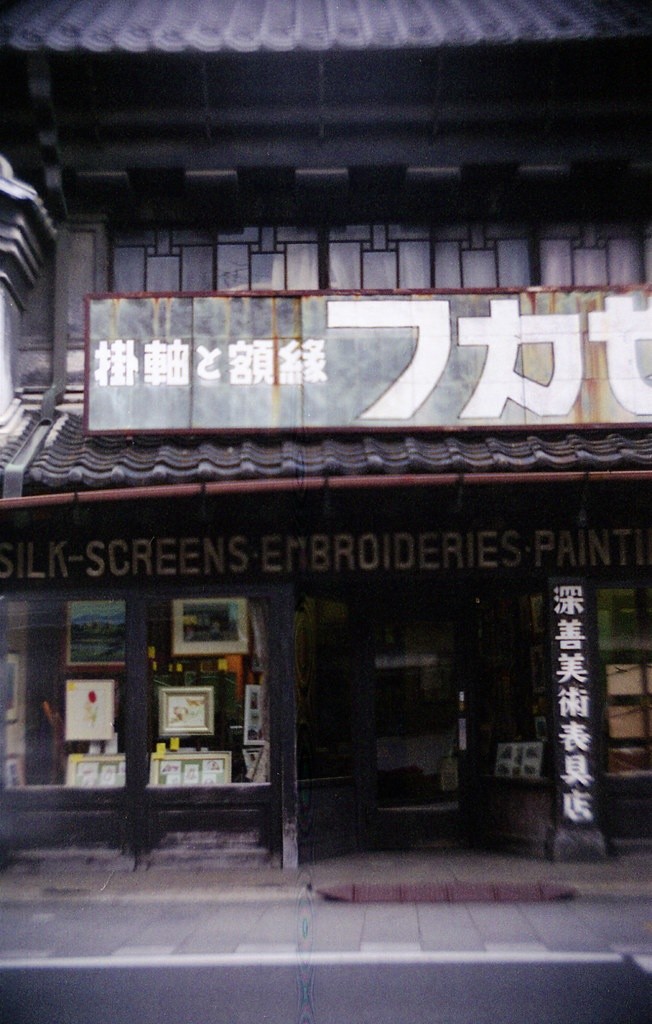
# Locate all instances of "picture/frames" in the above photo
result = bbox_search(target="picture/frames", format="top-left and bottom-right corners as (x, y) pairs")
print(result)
(171, 597), (250, 658)
(5, 753), (22, 786)
(66, 599), (126, 665)
(158, 686), (214, 735)
(244, 683), (265, 746)
(65, 679), (115, 743)
(243, 747), (268, 783)
(5, 648), (21, 724)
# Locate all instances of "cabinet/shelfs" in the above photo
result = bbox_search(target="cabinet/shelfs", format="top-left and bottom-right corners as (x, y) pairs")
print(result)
(148, 751), (232, 786)
(66, 753), (126, 788)
